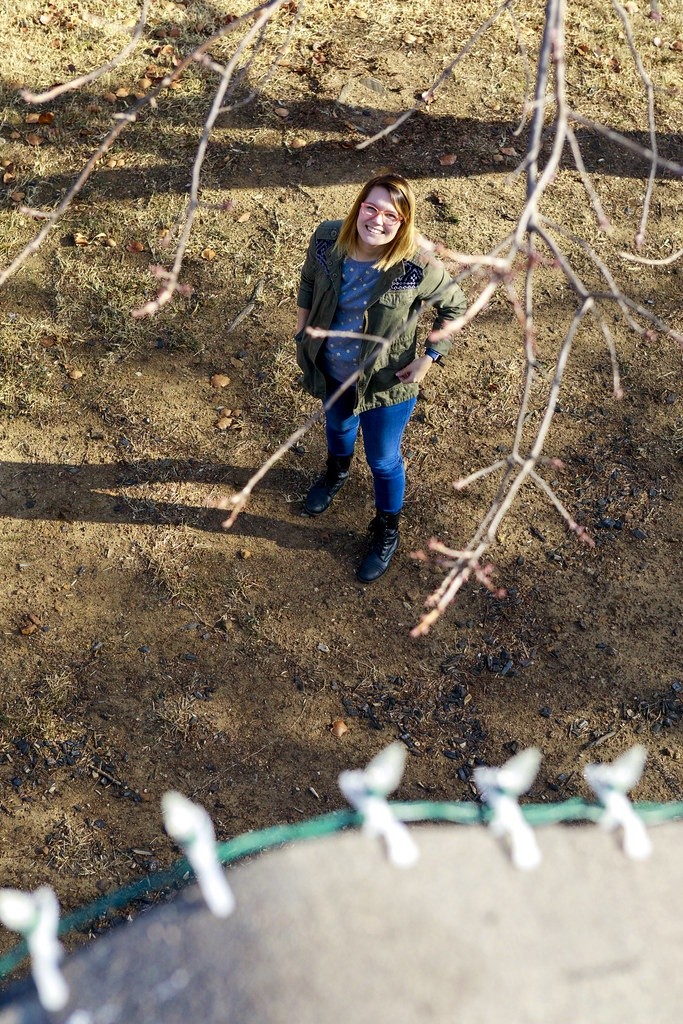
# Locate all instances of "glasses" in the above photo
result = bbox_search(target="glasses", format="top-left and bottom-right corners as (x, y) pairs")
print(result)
(361, 202), (403, 226)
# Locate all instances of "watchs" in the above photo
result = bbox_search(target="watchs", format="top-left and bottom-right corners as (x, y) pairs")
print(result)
(424, 348), (443, 362)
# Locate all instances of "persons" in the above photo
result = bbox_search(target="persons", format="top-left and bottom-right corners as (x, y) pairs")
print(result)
(295, 174), (468, 582)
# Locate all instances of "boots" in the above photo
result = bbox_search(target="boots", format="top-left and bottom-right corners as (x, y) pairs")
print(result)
(305, 450), (353, 515)
(357, 507), (401, 583)
